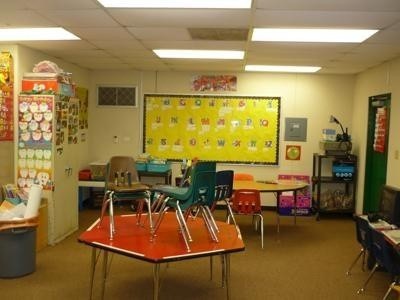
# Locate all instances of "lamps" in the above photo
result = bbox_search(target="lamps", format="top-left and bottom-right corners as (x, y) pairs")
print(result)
(329, 115), (350, 143)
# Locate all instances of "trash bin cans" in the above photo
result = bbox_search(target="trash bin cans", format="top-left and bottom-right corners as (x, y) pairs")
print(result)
(0, 227), (38, 279)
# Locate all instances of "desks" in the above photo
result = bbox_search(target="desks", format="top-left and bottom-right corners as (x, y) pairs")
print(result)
(232, 179), (311, 231)
(360, 213), (398, 229)
(78, 211), (246, 300)
(382, 230), (400, 250)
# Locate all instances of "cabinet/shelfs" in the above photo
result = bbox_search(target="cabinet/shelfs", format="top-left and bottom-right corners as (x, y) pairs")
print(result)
(17, 94), (81, 248)
(311, 153), (358, 222)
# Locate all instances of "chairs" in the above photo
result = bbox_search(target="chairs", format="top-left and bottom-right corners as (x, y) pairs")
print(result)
(344, 217), (377, 276)
(383, 256), (400, 300)
(355, 230), (400, 299)
(95, 156), (265, 253)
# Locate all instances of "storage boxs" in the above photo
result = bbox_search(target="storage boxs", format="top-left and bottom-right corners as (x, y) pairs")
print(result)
(319, 141), (349, 156)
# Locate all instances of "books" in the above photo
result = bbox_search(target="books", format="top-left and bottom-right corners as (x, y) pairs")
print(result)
(381, 230), (400, 246)
(322, 129), (336, 142)
(136, 154), (168, 166)
